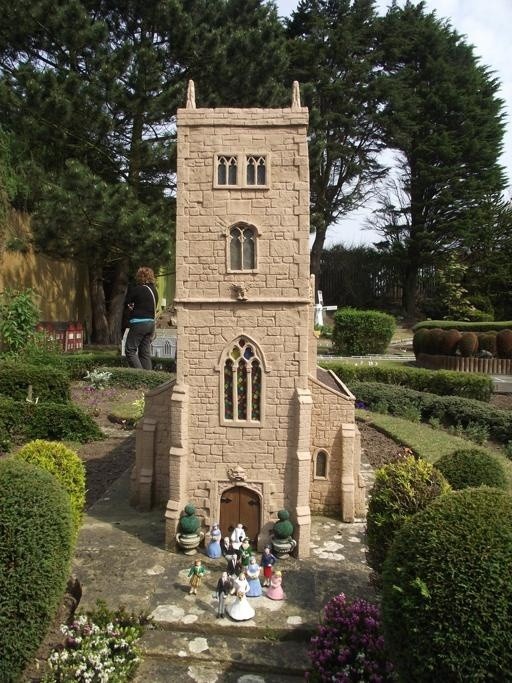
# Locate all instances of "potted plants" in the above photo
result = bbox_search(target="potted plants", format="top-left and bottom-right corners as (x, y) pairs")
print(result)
(176, 504), (205, 555)
(270, 509), (297, 560)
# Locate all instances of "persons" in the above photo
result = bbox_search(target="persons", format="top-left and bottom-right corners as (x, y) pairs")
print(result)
(225, 572), (256, 621)
(239, 538), (252, 567)
(230, 522), (246, 551)
(261, 542), (276, 586)
(215, 571), (232, 618)
(244, 554), (263, 596)
(223, 536), (242, 567)
(266, 568), (289, 599)
(121, 265), (159, 370)
(186, 557), (205, 595)
(208, 523), (222, 558)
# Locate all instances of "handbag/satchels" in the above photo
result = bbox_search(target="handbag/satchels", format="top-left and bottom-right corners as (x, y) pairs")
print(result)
(150, 321), (157, 341)
